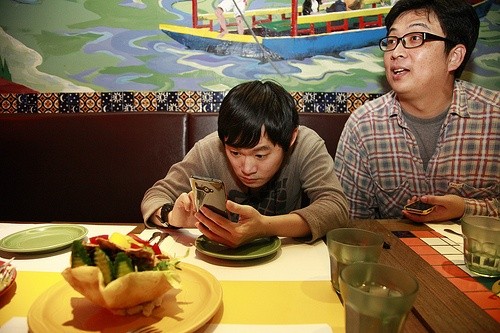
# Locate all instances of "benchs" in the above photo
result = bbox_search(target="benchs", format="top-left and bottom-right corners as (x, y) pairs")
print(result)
(0, 112), (351, 221)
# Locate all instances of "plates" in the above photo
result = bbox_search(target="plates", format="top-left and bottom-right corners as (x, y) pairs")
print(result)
(28, 261), (223, 333)
(0, 261), (17, 297)
(0, 225), (88, 255)
(195, 234), (282, 261)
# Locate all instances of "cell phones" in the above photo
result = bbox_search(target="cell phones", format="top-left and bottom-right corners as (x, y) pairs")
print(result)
(189, 176), (230, 221)
(403, 199), (436, 215)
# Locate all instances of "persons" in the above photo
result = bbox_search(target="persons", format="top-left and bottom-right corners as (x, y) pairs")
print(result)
(141, 81), (350, 248)
(334, 0), (500, 221)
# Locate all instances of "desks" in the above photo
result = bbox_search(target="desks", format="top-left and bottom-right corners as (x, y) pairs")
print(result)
(0, 219), (500, 333)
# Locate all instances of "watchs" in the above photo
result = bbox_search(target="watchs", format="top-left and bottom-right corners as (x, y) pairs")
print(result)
(155, 203), (183, 230)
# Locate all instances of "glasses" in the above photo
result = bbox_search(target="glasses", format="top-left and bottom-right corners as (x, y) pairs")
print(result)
(379, 32), (455, 52)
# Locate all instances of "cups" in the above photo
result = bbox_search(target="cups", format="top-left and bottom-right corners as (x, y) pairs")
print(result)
(327, 228), (384, 294)
(461, 216), (500, 277)
(339, 263), (419, 333)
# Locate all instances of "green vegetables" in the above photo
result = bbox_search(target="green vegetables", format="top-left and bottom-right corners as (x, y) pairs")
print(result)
(141, 260), (182, 273)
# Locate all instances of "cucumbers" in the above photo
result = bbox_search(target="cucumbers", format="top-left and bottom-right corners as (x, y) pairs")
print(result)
(70, 241), (136, 288)
